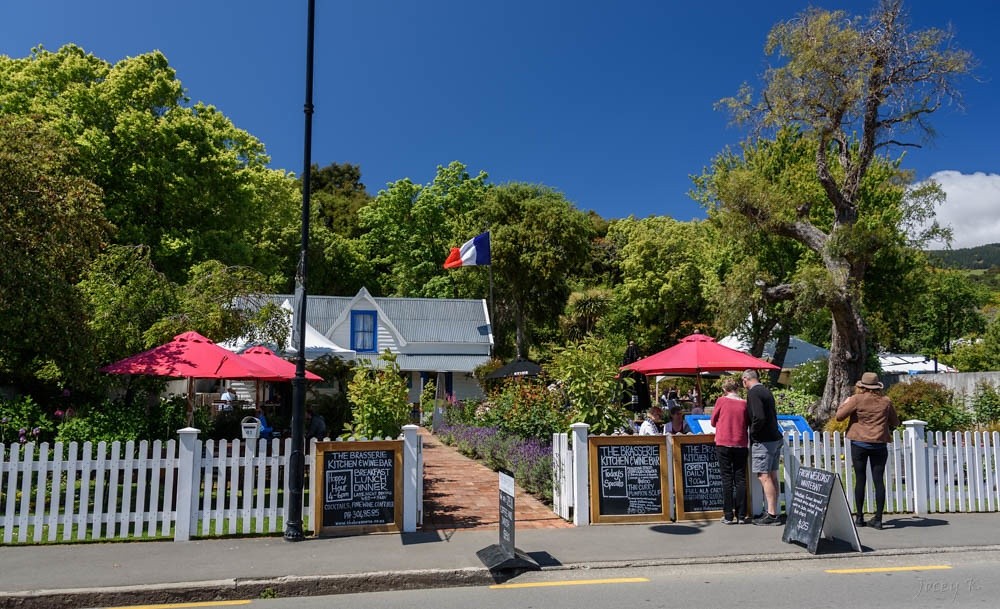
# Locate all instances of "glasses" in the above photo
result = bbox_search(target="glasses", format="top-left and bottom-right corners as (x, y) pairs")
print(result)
(657, 414), (661, 419)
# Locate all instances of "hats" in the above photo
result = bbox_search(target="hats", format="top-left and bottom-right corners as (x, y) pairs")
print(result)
(856, 372), (884, 389)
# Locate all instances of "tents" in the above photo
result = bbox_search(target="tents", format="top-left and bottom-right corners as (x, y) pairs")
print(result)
(654, 308), (838, 406)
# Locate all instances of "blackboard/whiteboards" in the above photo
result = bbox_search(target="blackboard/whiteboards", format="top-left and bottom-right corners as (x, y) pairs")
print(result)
(588, 435), (672, 524)
(313, 440), (405, 537)
(783, 466), (863, 555)
(497, 489), (516, 558)
(670, 434), (751, 520)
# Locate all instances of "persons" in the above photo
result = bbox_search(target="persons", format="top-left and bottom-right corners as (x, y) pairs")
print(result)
(253, 408), (279, 441)
(304, 406), (326, 440)
(836, 372), (901, 530)
(663, 407), (690, 434)
(661, 386), (696, 407)
(639, 407), (663, 436)
(742, 368), (785, 526)
(710, 379), (749, 524)
(218, 385), (237, 411)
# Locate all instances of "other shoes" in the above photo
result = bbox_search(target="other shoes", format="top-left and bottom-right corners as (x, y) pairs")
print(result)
(736, 519), (745, 524)
(721, 516), (733, 524)
(869, 518), (882, 530)
(855, 514), (865, 527)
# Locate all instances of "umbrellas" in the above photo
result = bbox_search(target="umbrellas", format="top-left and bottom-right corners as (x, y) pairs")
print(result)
(99, 328), (282, 427)
(616, 328), (781, 410)
(228, 343), (324, 409)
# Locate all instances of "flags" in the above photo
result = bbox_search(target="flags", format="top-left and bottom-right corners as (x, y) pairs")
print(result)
(443, 231), (492, 268)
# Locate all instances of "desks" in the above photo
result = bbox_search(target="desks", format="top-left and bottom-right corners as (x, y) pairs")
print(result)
(264, 403), (280, 414)
(212, 402), (252, 410)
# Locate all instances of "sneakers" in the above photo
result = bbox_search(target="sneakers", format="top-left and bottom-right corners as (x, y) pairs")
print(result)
(752, 513), (781, 526)
